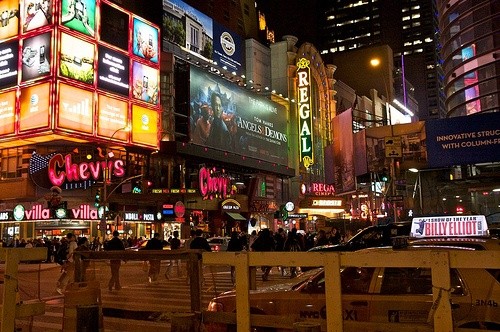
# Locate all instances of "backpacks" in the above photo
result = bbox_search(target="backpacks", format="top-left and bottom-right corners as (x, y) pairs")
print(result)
(56, 245), (67, 264)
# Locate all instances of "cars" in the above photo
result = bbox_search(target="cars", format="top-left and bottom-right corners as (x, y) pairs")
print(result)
(290, 220), (412, 278)
(204, 235), (500, 332)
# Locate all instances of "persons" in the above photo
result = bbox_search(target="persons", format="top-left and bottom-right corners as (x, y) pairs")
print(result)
(164, 231), (184, 279)
(122, 236), (143, 249)
(227, 228), (352, 287)
(191, 229), (211, 287)
(0, 233), (103, 294)
(106, 230), (127, 291)
(147, 233), (165, 283)
(191, 91), (243, 149)
(184, 230), (196, 286)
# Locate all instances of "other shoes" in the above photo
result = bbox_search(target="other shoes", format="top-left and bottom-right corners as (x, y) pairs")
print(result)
(57, 289), (63, 294)
(108, 285), (113, 290)
(117, 285), (121, 290)
(79, 283), (87, 288)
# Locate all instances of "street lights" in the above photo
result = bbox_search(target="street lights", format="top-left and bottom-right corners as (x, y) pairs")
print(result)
(103, 125), (131, 202)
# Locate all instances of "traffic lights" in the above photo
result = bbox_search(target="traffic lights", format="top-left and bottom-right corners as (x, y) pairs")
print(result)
(93, 186), (104, 208)
(381, 173), (390, 183)
